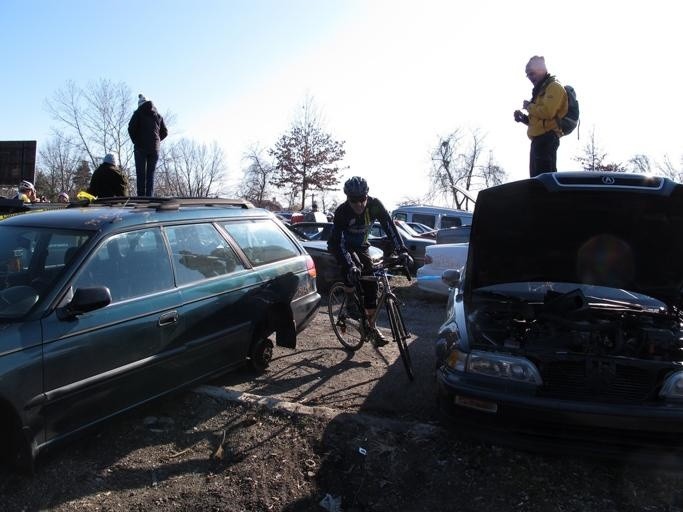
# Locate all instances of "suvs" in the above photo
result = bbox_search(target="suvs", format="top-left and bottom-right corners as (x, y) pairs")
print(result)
(0, 196), (323, 487)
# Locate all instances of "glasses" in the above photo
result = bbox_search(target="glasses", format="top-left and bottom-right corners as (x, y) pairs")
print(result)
(349, 197), (366, 203)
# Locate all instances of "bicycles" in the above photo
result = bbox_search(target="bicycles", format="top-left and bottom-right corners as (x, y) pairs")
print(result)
(329, 256), (420, 382)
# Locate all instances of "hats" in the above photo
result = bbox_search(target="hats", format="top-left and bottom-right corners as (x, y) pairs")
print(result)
(526, 56), (546, 73)
(138, 95), (148, 106)
(104, 154), (115, 165)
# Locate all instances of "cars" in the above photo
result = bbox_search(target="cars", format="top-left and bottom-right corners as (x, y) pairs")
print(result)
(275, 204), (472, 300)
(436, 171), (682, 445)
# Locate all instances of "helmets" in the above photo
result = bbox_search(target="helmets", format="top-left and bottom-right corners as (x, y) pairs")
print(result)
(344, 176), (369, 196)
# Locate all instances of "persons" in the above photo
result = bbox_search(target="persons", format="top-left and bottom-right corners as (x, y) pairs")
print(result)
(514, 55), (569, 179)
(127, 94), (168, 198)
(13, 179), (37, 203)
(58, 192), (69, 203)
(87, 153), (130, 199)
(327, 175), (410, 346)
(311, 204), (317, 212)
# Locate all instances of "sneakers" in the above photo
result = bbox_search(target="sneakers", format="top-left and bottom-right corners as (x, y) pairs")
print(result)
(374, 329), (389, 347)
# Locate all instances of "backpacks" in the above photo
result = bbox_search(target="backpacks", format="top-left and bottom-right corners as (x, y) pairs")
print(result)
(555, 85), (579, 134)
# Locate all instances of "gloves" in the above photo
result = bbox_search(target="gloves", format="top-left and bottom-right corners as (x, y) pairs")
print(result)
(348, 267), (361, 282)
(401, 252), (414, 269)
